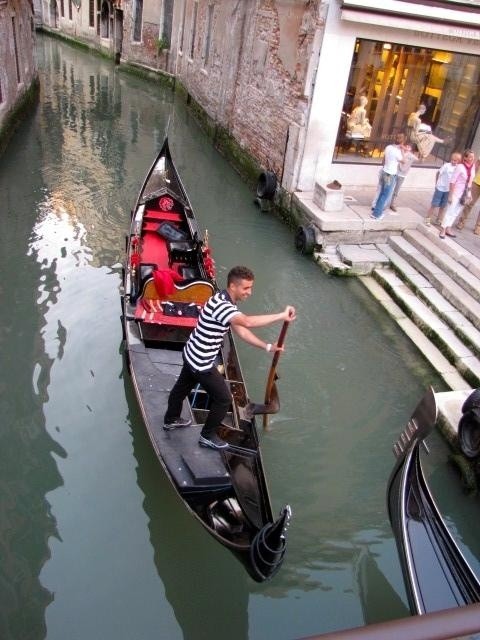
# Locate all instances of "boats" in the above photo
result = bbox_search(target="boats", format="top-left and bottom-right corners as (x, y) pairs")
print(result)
(117, 131), (293, 586)
(384, 382), (479, 614)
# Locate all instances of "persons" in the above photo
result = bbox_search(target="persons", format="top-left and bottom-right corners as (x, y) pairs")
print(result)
(161, 265), (297, 451)
(345, 96), (373, 139)
(424, 152), (462, 229)
(406, 105), (451, 159)
(370, 133), (407, 220)
(439, 149), (475, 238)
(389, 144), (425, 213)
(456, 160), (480, 235)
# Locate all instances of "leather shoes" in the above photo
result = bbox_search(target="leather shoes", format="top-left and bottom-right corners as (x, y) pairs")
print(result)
(439, 226), (457, 240)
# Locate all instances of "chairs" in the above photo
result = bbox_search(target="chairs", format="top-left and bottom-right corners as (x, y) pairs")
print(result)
(336, 112), (451, 165)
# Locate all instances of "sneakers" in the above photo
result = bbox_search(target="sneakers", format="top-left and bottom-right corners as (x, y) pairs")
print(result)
(424, 216), (444, 228)
(369, 204), (399, 220)
(457, 221), (466, 229)
(473, 222), (480, 235)
(163, 417), (193, 431)
(198, 431), (230, 451)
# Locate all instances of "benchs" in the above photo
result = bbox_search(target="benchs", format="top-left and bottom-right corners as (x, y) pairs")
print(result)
(132, 276), (215, 330)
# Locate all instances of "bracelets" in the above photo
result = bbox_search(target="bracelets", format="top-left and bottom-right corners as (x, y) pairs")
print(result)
(265, 343), (272, 352)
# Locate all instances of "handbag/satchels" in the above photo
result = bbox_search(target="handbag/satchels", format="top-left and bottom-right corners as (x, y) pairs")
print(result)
(459, 187), (473, 206)
